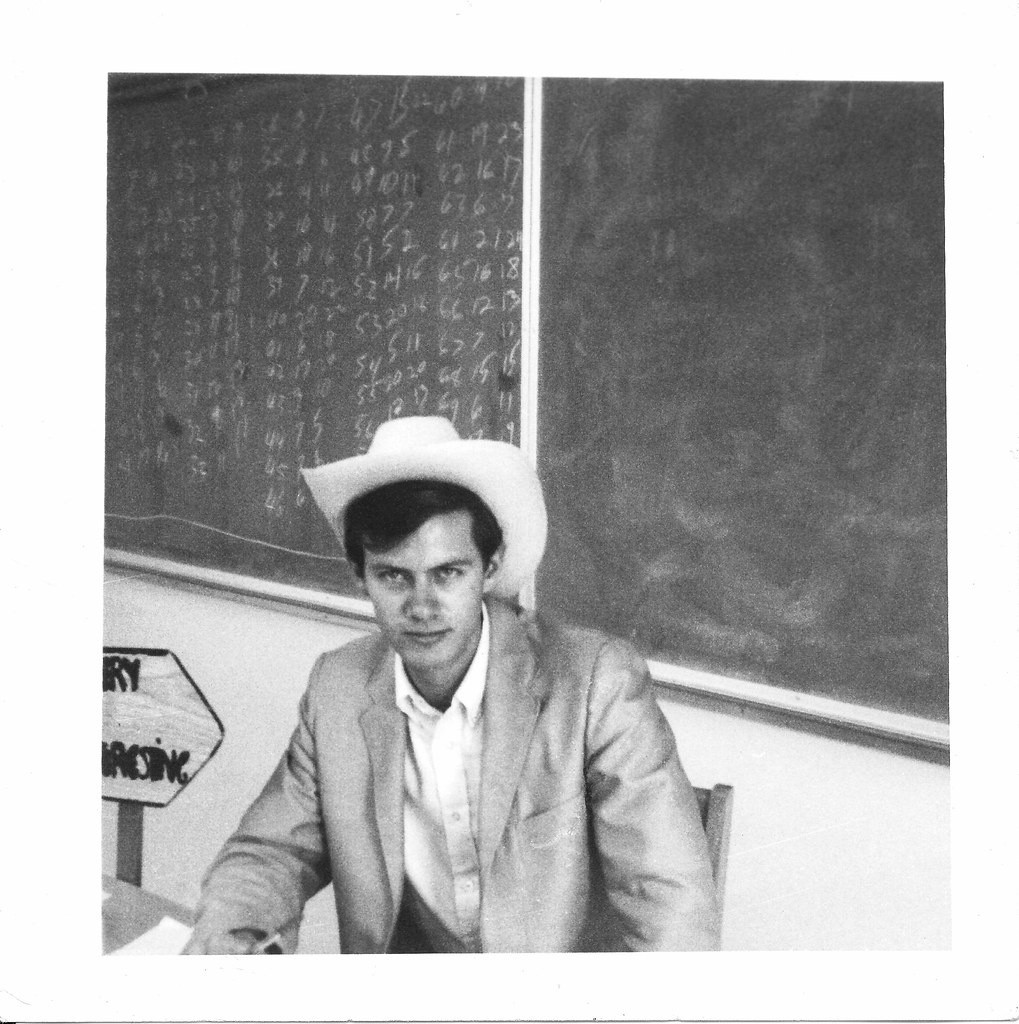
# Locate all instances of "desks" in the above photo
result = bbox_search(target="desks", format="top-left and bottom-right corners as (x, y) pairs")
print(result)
(102, 876), (198, 956)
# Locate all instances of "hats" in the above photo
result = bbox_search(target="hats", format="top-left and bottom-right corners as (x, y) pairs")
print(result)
(299, 414), (548, 600)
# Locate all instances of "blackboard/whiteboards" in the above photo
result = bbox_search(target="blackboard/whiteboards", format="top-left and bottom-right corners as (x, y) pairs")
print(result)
(103, 72), (952, 768)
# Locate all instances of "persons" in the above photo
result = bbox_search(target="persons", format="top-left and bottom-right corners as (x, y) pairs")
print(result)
(179, 415), (721, 953)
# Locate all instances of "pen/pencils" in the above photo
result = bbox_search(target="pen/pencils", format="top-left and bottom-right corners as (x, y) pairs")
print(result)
(248, 911), (305, 954)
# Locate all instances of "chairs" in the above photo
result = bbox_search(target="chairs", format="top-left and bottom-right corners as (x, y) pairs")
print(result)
(692, 784), (733, 908)
